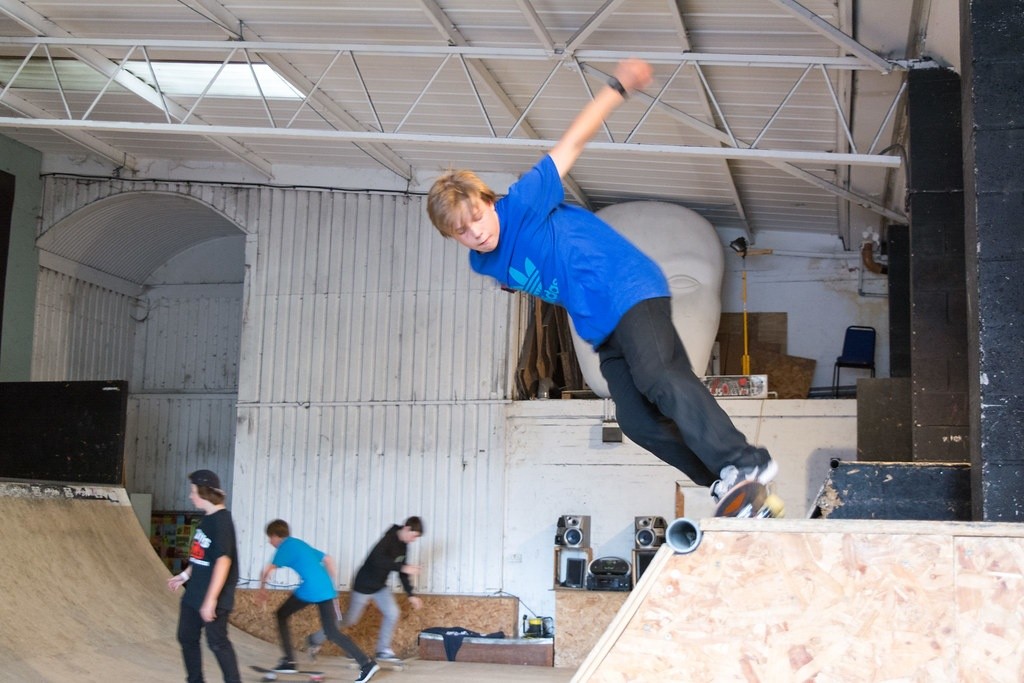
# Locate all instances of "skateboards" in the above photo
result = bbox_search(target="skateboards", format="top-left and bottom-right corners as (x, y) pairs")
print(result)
(711, 478), (793, 519)
(248, 659), (324, 683)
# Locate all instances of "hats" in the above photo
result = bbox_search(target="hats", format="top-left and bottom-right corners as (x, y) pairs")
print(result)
(188, 470), (226, 496)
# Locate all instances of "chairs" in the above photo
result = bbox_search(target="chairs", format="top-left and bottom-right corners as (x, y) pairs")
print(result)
(831, 326), (877, 399)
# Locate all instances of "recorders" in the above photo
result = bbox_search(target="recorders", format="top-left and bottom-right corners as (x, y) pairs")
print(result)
(587, 556), (632, 577)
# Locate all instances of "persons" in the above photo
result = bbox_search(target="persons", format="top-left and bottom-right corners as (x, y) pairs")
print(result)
(428, 61), (780, 498)
(168, 469), (242, 683)
(307, 516), (423, 662)
(256, 519), (382, 683)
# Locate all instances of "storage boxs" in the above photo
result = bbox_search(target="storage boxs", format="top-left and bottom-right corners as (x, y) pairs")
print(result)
(553, 546), (592, 590)
(418, 626), (554, 667)
(632, 548), (660, 591)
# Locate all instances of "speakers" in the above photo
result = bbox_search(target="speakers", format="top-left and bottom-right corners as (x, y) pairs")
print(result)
(635, 516), (667, 551)
(554, 516), (590, 548)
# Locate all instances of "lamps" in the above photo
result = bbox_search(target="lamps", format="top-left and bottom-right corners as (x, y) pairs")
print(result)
(729, 237), (748, 258)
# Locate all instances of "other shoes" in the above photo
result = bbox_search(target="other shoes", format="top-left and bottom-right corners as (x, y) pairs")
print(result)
(304, 634), (319, 665)
(710, 458), (777, 503)
(375, 651), (401, 662)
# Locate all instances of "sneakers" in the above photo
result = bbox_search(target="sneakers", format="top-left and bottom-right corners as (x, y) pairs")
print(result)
(272, 661), (299, 674)
(355, 660), (379, 683)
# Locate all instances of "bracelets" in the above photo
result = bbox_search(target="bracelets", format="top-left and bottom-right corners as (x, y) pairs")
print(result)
(179, 572), (190, 581)
(605, 78), (629, 100)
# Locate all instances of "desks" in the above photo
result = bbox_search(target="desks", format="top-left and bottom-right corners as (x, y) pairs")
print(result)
(548, 588), (631, 668)
